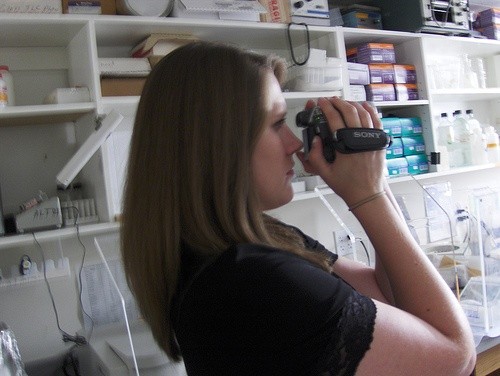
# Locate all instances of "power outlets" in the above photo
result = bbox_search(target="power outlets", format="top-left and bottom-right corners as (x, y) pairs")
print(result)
(333, 229), (354, 257)
(455, 206), (469, 224)
(0, 258), (71, 287)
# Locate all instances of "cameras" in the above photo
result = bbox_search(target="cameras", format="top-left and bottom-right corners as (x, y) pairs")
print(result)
(296, 102), (347, 163)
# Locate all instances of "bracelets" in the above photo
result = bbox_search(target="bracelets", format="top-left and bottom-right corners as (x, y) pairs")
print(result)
(348, 190), (387, 212)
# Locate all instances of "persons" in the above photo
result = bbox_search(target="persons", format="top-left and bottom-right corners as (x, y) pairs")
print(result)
(118, 39), (478, 376)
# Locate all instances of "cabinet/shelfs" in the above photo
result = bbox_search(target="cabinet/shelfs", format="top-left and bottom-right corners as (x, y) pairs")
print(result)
(1, 13), (500, 250)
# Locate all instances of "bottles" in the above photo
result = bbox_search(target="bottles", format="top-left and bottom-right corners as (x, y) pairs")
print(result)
(483, 126), (500, 162)
(0, 73), (8, 106)
(453, 110), (476, 165)
(0, 66), (14, 106)
(439, 113), (453, 164)
(466, 110), (482, 163)
